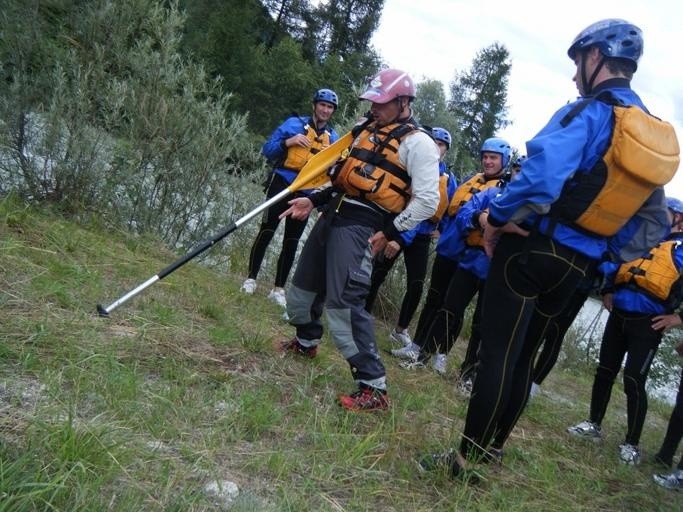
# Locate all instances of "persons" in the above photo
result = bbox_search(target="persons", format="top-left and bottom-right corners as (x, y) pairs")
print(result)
(364, 128), (458, 346)
(239, 88), (341, 311)
(411, 18), (681, 488)
(564, 198), (683, 469)
(387, 137), (512, 377)
(392, 155), (532, 397)
(278, 68), (440, 414)
(653, 339), (683, 492)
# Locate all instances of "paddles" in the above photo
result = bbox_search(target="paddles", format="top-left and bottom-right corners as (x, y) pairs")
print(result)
(95, 131), (355, 319)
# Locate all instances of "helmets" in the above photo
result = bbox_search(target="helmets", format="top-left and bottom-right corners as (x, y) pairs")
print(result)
(481, 138), (511, 175)
(566, 18), (643, 91)
(513, 155), (527, 166)
(314, 89), (339, 106)
(431, 126), (452, 149)
(666, 196), (683, 213)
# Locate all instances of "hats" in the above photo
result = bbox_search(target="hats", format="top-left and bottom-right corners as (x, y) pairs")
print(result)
(358, 68), (416, 103)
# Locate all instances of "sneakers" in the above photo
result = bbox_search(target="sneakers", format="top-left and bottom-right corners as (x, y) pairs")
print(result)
(271, 336), (318, 358)
(455, 379), (473, 397)
(390, 328), (411, 346)
(529, 383), (539, 397)
(398, 355), (427, 370)
(239, 279), (257, 295)
(415, 448), (483, 486)
(432, 351), (448, 374)
(337, 384), (390, 413)
(267, 288), (287, 308)
(391, 342), (419, 360)
(481, 448), (503, 466)
(652, 471), (682, 491)
(619, 442), (639, 466)
(566, 421), (603, 440)
